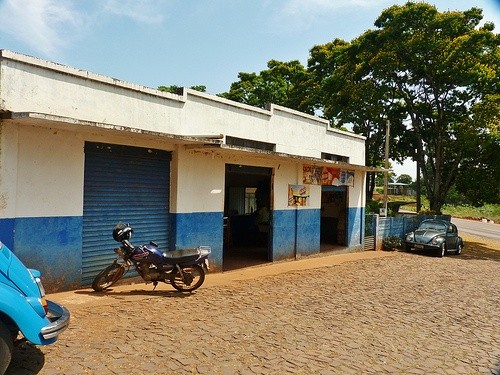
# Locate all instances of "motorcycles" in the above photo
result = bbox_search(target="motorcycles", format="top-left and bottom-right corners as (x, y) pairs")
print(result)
(92, 239), (212, 293)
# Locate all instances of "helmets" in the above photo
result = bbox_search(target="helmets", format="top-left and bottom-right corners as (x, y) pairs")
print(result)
(113, 221), (131, 242)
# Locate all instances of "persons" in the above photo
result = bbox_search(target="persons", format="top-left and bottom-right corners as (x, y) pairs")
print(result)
(255, 198), (270, 231)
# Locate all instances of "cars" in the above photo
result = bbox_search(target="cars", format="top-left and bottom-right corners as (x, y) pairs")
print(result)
(404, 218), (463, 257)
